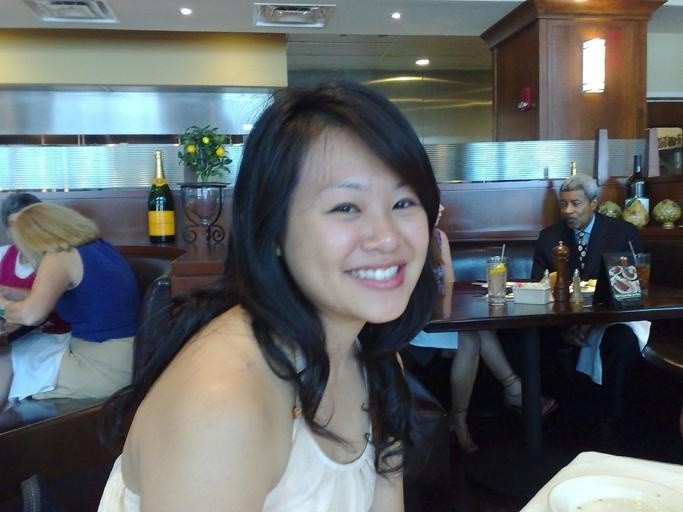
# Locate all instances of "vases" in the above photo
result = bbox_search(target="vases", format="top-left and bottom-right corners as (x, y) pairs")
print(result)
(177, 181), (227, 243)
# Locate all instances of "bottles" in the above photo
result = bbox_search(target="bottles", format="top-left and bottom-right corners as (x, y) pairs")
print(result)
(147, 150), (177, 244)
(569, 269), (586, 303)
(625, 154), (650, 217)
(543, 269), (555, 303)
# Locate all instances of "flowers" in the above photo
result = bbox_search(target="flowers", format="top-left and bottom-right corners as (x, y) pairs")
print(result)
(175, 124), (233, 183)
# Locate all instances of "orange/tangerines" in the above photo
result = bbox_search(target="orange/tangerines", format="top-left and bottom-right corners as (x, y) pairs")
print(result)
(186, 144), (196, 153)
(215, 145), (225, 157)
(202, 137), (209, 144)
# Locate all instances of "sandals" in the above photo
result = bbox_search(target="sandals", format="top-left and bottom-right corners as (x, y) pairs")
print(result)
(501, 371), (559, 418)
(441, 406), (480, 456)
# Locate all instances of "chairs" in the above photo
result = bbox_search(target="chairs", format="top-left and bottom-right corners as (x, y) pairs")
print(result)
(1, 257), (175, 510)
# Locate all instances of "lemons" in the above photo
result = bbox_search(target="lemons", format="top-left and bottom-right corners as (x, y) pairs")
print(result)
(489, 264), (506, 277)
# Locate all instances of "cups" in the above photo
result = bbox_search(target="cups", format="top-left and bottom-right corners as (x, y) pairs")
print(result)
(487, 256), (508, 306)
(634, 254), (651, 294)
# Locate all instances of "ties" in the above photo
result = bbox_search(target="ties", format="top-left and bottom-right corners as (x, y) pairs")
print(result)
(577, 232), (587, 269)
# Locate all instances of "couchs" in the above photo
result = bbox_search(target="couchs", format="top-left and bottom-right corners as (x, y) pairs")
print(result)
(396, 237), (681, 409)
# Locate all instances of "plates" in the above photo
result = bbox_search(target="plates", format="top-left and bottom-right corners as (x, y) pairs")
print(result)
(610, 265), (637, 296)
(569, 281), (596, 297)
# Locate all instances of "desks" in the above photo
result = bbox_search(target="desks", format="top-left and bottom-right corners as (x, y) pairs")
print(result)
(2, 308), (53, 346)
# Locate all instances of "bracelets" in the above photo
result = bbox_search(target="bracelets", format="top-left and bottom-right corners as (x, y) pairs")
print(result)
(0, 299), (14, 319)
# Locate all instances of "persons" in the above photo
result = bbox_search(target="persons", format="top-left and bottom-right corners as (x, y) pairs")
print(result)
(529, 172), (652, 441)
(0, 192), (71, 329)
(0, 201), (143, 416)
(94, 78), (438, 512)
(400, 203), (561, 457)
